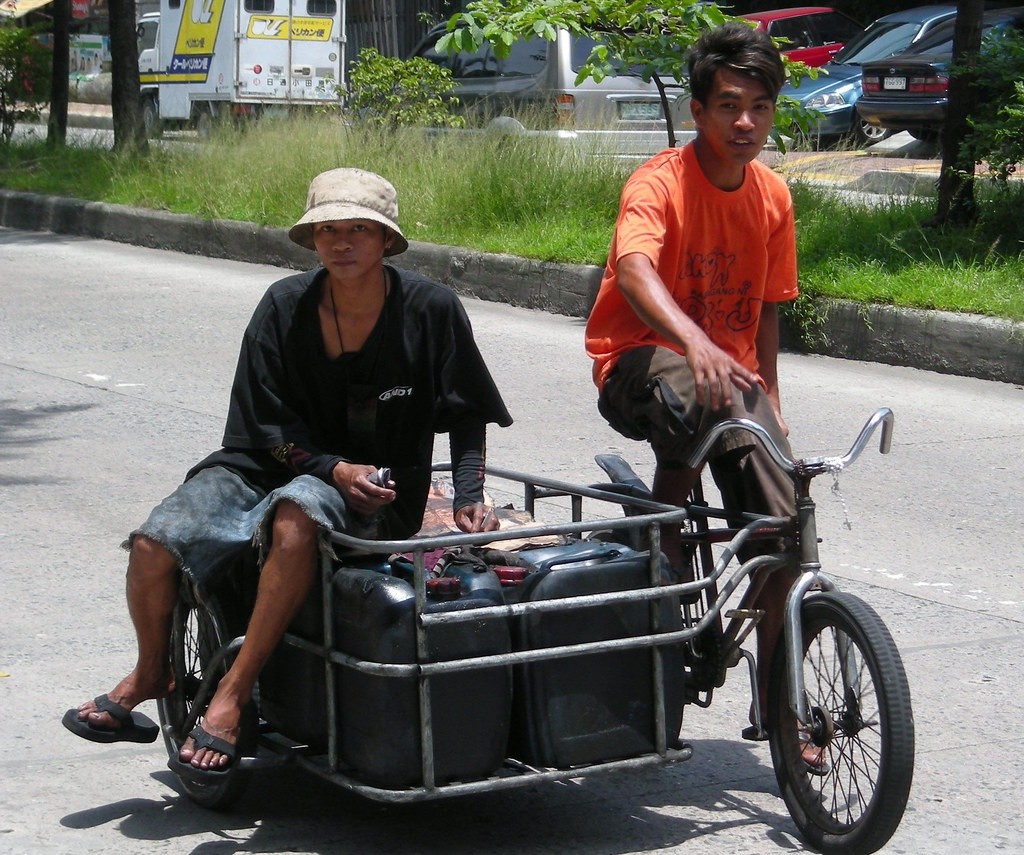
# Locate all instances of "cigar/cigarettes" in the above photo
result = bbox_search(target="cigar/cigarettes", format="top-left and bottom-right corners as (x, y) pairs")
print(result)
(478, 508), (493, 531)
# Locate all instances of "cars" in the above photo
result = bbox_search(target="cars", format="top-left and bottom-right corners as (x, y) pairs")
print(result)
(725, 0), (1024, 151)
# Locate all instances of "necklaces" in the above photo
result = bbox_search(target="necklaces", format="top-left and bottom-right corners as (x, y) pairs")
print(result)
(330, 288), (344, 353)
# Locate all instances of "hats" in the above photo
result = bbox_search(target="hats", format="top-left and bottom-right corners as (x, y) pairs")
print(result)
(289, 168), (408, 257)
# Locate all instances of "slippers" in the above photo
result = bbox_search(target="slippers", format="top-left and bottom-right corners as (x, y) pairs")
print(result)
(742, 725), (831, 776)
(167, 726), (246, 785)
(62, 694), (159, 743)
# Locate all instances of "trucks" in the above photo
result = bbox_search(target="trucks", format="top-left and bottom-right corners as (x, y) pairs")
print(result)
(137, 0), (347, 144)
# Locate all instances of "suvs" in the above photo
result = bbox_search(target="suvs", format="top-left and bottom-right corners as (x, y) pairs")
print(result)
(355, 14), (695, 131)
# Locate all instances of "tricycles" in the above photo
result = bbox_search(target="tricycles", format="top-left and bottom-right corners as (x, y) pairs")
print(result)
(158, 406), (917, 855)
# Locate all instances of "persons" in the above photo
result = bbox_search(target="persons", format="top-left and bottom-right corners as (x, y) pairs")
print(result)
(586, 24), (830, 776)
(63, 168), (514, 786)
(71, 51), (99, 72)
